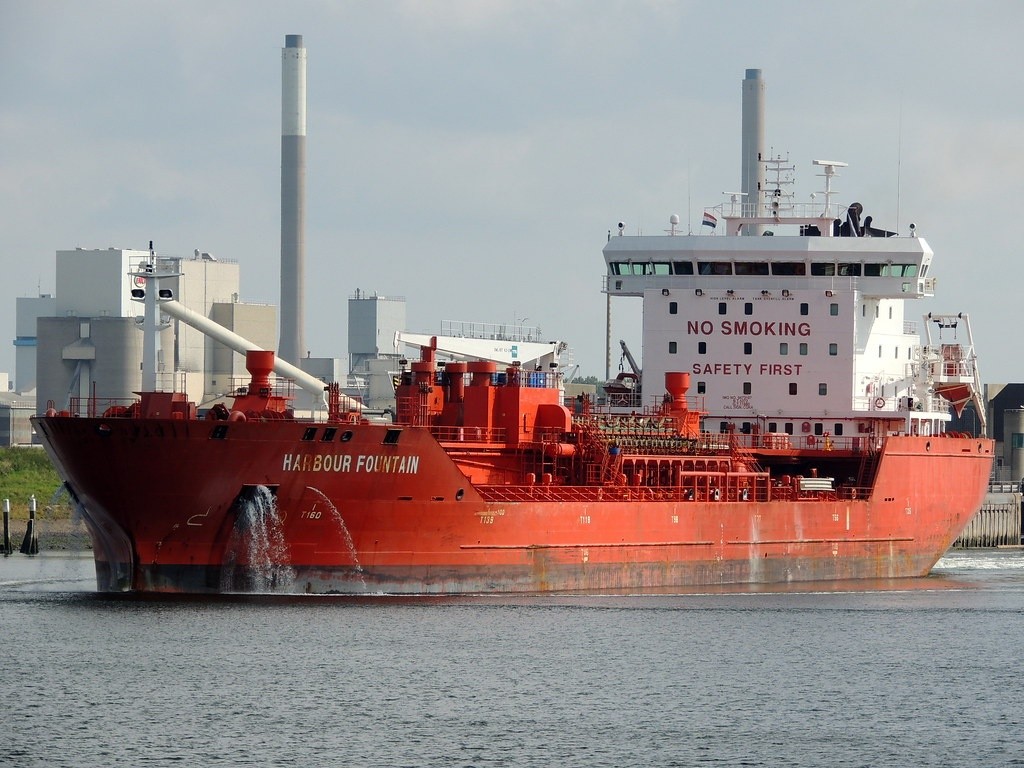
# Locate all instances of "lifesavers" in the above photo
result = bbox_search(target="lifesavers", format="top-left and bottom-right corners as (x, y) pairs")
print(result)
(807, 435), (816, 446)
(852, 489), (856, 499)
(875, 398), (885, 408)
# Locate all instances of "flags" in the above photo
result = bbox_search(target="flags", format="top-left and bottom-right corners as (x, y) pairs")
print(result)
(702, 212), (717, 228)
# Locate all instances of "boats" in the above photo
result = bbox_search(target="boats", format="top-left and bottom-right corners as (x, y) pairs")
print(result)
(931, 345), (975, 419)
(27, 34), (996, 595)
